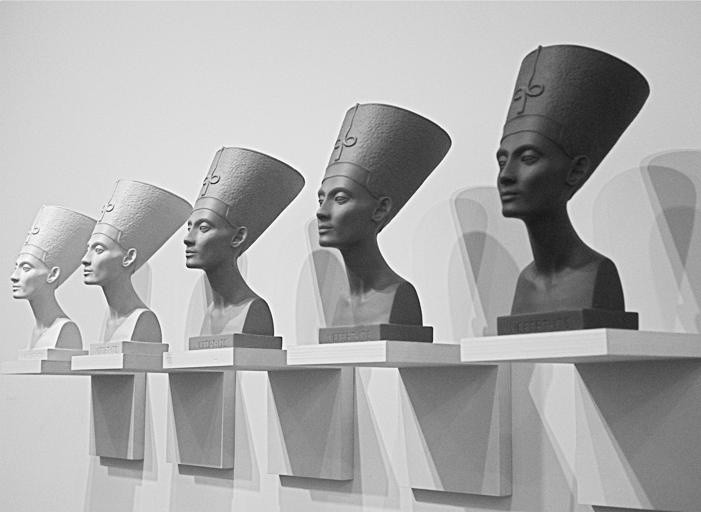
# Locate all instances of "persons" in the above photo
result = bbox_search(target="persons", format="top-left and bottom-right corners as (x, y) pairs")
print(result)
(181, 208), (276, 336)
(315, 175), (423, 326)
(10, 254), (98, 350)
(81, 233), (164, 342)
(496, 132), (625, 312)
